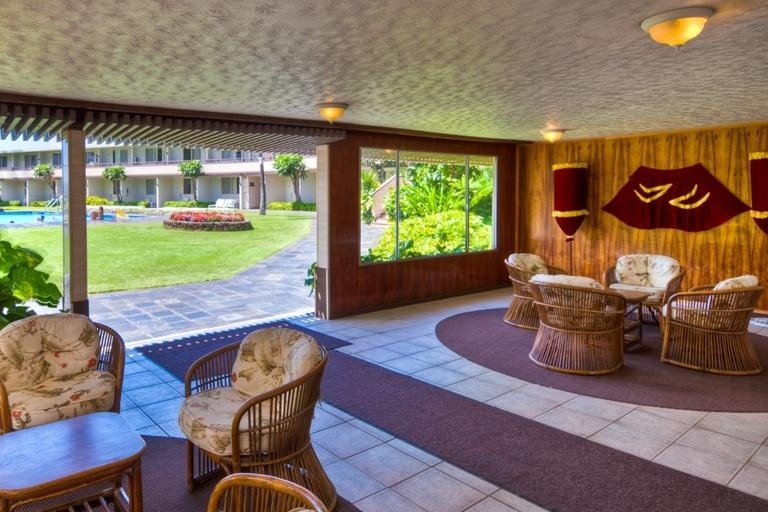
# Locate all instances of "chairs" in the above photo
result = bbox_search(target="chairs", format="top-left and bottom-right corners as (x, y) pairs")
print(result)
(0, 314), (125, 437)
(659, 275), (764, 376)
(605, 252), (685, 327)
(178, 328), (337, 511)
(208, 473), (329, 511)
(502, 252), (568, 331)
(528, 274), (627, 375)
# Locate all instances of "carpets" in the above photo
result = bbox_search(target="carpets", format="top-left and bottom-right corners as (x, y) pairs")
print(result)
(134, 319), (354, 385)
(262, 350), (768, 512)
(435, 307), (768, 413)
(0, 436), (360, 510)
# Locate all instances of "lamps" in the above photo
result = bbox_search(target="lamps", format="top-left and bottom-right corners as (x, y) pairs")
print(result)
(640, 6), (716, 53)
(315, 103), (348, 126)
(540, 128), (567, 145)
